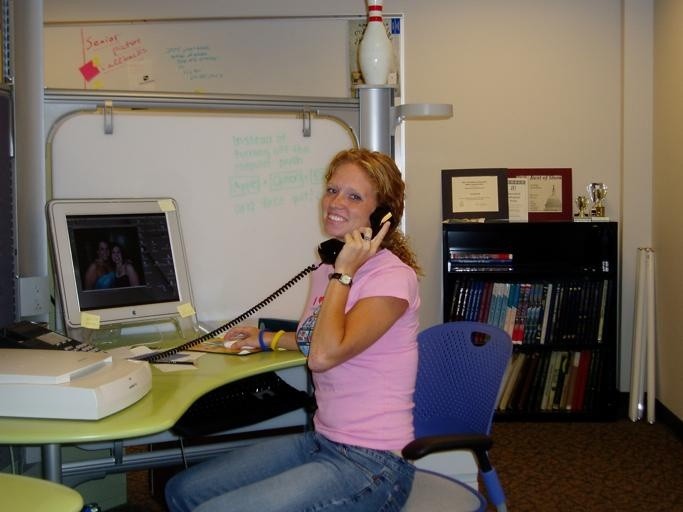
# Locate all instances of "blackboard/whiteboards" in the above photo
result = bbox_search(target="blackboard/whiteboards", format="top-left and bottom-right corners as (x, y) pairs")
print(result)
(44, 13), (405, 234)
(43, 88), (362, 451)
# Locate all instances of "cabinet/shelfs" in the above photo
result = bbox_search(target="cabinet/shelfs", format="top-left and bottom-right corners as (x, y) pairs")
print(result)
(443, 222), (617, 422)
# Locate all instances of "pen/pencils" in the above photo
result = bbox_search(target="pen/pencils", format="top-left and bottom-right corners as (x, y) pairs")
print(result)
(153, 361), (194, 364)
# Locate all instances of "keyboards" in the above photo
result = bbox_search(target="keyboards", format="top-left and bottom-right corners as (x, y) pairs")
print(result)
(167, 372), (307, 440)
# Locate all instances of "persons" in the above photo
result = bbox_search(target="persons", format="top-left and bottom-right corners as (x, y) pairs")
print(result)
(163, 148), (423, 512)
(83, 240), (140, 291)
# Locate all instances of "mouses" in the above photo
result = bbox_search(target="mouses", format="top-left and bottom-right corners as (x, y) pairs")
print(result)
(223, 337), (258, 356)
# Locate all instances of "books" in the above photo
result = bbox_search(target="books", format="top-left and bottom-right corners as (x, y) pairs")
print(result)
(444, 244), (617, 416)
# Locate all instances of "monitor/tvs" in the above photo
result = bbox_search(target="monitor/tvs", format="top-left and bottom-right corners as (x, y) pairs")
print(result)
(44, 198), (196, 343)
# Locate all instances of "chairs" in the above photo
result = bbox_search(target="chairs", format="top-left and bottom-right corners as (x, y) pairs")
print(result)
(402, 321), (512, 512)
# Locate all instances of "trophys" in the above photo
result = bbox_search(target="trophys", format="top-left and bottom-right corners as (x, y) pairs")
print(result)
(575, 180), (609, 222)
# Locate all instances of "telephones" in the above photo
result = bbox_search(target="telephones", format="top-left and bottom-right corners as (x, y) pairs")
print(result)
(317, 203), (398, 264)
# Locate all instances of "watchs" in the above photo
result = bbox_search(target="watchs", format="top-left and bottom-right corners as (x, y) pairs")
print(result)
(328, 272), (355, 289)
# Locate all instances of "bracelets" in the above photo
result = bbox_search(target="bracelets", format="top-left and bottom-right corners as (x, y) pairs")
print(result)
(260, 327), (268, 353)
(270, 329), (285, 351)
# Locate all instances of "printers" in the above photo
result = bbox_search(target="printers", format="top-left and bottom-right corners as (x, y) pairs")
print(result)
(0, 349), (158, 419)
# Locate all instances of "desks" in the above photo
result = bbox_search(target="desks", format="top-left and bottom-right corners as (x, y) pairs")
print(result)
(0, 331), (308, 489)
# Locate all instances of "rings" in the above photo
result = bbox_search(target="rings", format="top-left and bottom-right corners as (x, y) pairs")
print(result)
(364, 237), (373, 242)
